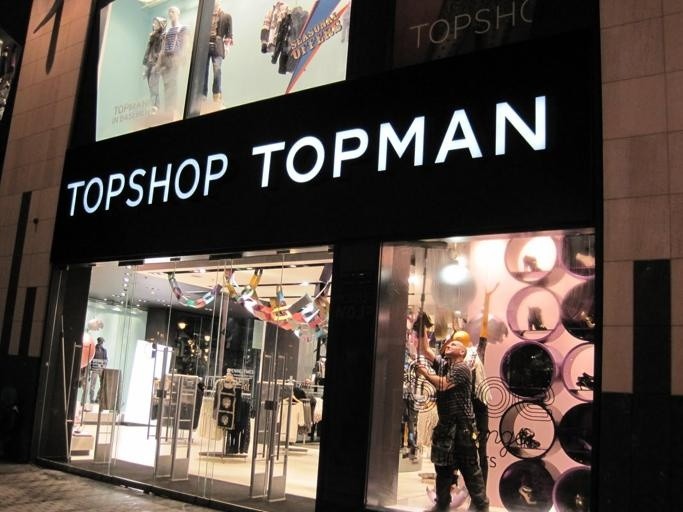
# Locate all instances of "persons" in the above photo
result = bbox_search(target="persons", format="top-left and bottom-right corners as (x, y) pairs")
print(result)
(142, 6), (189, 117)
(413, 306), (489, 511)
(201, 0), (234, 104)
(88, 336), (108, 404)
(400, 329), (420, 462)
(443, 279), (501, 511)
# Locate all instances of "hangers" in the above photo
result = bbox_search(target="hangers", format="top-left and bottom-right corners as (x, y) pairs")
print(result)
(279, 392), (301, 405)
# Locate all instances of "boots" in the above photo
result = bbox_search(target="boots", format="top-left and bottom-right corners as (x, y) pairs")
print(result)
(422, 494), (451, 512)
(467, 499), (489, 512)
